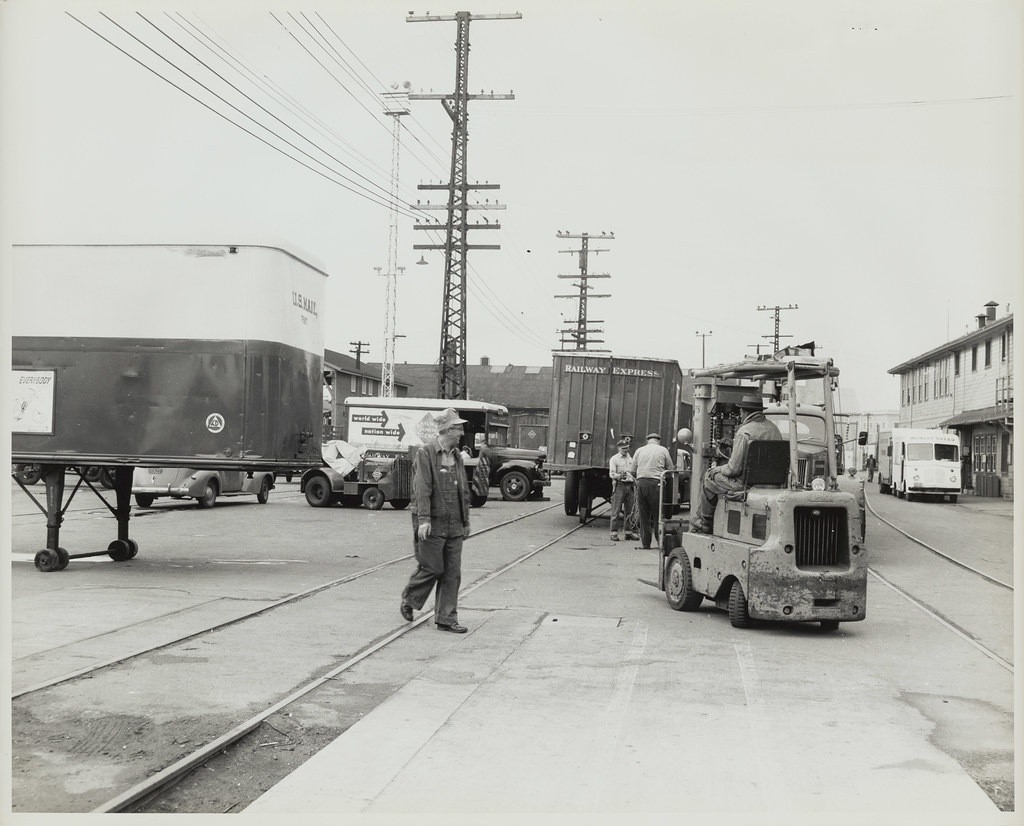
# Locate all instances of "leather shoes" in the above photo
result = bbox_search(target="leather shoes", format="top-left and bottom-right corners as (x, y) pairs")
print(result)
(401, 595), (413, 622)
(438, 620), (468, 632)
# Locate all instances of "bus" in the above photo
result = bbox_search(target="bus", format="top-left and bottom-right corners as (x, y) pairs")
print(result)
(761, 400), (845, 492)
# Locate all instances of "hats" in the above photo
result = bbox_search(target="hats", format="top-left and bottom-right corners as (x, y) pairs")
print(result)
(617, 440), (631, 449)
(646, 433), (662, 440)
(735, 394), (768, 411)
(434, 408), (468, 435)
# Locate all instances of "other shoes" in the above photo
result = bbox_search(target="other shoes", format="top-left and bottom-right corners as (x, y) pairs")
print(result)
(690, 516), (711, 533)
(611, 534), (620, 542)
(625, 532), (640, 541)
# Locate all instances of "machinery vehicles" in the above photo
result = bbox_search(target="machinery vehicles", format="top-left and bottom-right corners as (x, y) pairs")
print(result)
(636, 354), (869, 631)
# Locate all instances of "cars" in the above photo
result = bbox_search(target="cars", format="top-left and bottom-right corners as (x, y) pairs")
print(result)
(131, 464), (277, 509)
(15, 463), (118, 491)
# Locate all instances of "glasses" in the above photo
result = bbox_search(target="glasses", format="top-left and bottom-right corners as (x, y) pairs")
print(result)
(449, 426), (465, 431)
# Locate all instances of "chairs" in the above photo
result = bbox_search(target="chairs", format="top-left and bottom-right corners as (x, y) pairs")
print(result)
(719, 440), (791, 502)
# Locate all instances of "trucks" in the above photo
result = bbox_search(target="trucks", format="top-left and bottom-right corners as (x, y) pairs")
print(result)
(545, 349), (695, 519)
(876, 427), (969, 504)
(344, 394), (552, 503)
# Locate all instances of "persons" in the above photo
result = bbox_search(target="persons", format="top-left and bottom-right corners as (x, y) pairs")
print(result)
(460, 445), (473, 459)
(609, 440), (640, 541)
(630, 433), (675, 549)
(399, 407), (472, 633)
(690, 393), (782, 534)
(865, 454), (876, 482)
